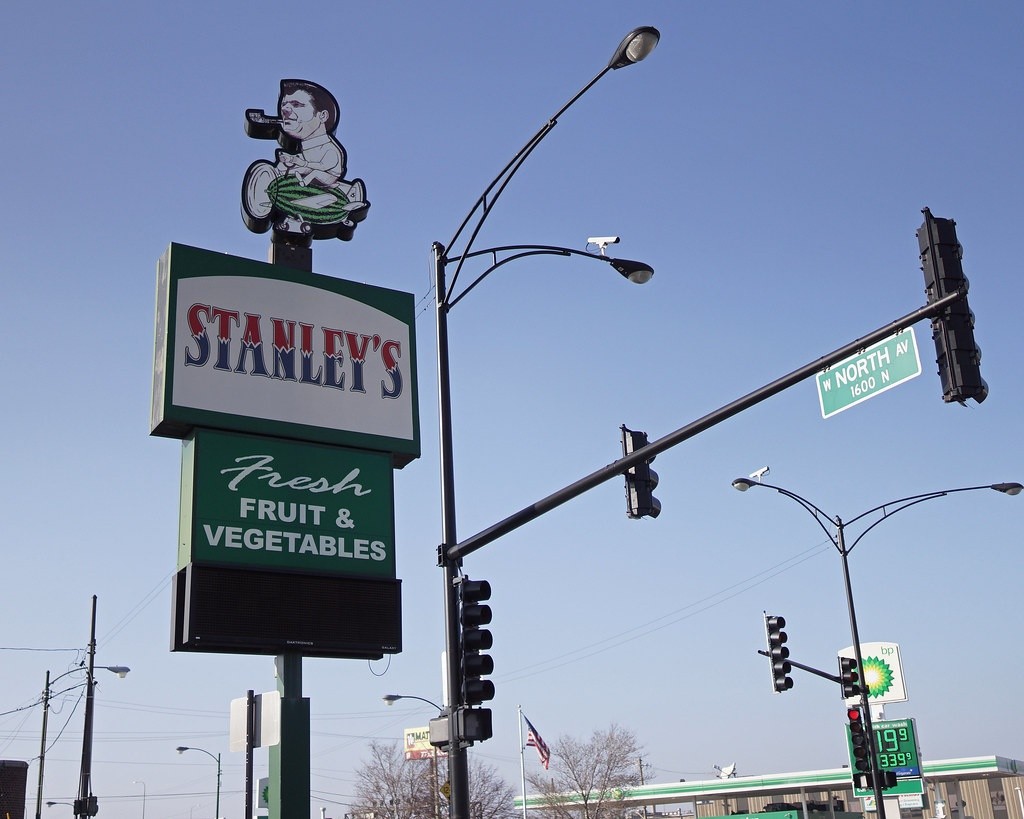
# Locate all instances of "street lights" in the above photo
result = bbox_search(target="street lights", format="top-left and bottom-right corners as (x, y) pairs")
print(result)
(730, 478), (1024, 819)
(176, 747), (221, 819)
(36, 665), (130, 819)
(433, 27), (659, 817)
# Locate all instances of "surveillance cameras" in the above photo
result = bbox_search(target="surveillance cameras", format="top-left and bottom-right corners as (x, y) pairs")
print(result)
(587, 235), (623, 256)
(747, 465), (771, 480)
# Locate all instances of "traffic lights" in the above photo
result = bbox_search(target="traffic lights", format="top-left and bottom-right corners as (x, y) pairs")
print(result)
(839, 657), (860, 699)
(766, 615), (793, 691)
(853, 773), (871, 787)
(847, 707), (869, 772)
(621, 427), (661, 519)
(456, 707), (493, 740)
(461, 578), (495, 703)
